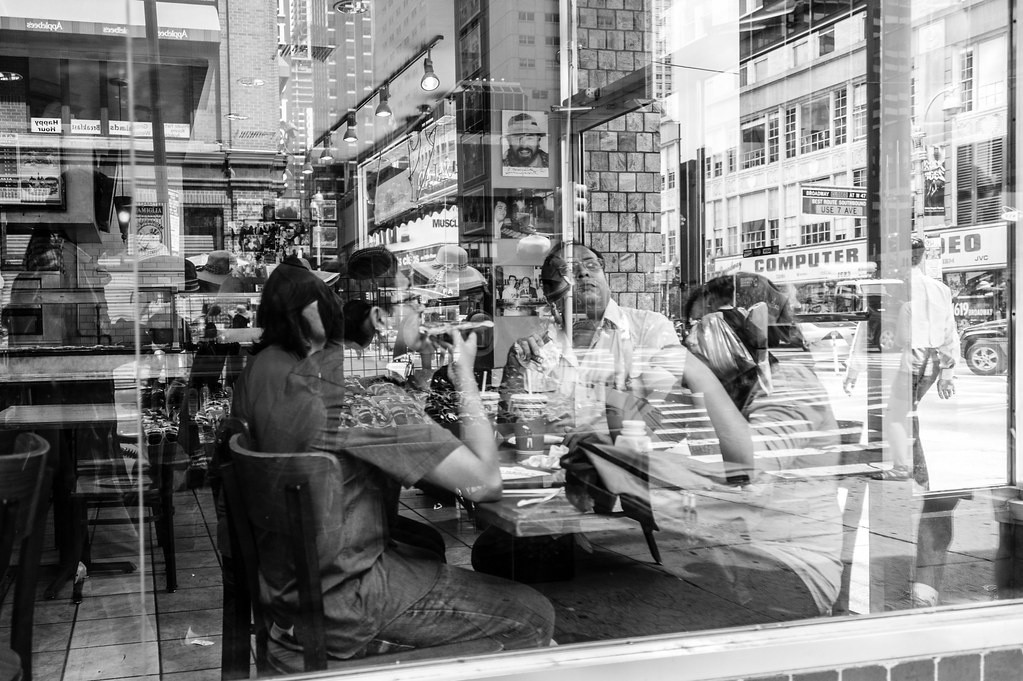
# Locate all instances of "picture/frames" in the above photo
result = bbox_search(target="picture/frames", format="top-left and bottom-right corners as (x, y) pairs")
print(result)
(457, 23), (485, 85)
(501, 109), (550, 178)
(0, 147), (68, 212)
(461, 75), (485, 138)
(496, 264), (553, 318)
(461, 181), (489, 237)
(493, 197), (554, 267)
(454, 1), (484, 34)
(460, 127), (485, 189)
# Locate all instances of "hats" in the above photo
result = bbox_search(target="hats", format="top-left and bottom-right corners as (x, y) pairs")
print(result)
(258, 258), (340, 316)
(493, 235), (570, 302)
(503, 112), (547, 137)
(460, 310), (498, 358)
(407, 244), (486, 292)
(185, 251), (250, 285)
(137, 255), (199, 294)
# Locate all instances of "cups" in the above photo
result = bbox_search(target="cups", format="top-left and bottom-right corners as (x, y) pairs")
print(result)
(480, 390), (500, 426)
(510, 394), (548, 454)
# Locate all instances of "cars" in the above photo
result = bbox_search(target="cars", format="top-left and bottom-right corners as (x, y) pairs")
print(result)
(958, 315), (1013, 376)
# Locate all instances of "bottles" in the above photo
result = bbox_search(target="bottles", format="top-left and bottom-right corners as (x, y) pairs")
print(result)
(614, 421), (652, 452)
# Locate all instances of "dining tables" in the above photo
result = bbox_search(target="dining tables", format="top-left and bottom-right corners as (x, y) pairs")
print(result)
(436, 429), (693, 541)
(0, 403), (141, 604)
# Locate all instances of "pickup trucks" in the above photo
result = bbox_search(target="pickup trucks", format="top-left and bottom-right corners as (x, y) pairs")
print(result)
(791, 290), (910, 355)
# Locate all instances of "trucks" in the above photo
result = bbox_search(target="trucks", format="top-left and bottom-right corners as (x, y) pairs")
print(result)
(832, 277), (909, 315)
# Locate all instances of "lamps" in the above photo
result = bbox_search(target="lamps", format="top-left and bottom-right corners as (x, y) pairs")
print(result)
(421, 49), (440, 91)
(343, 112), (358, 142)
(375, 88), (392, 117)
(108, 78), (133, 246)
(301, 156), (314, 174)
(319, 137), (333, 160)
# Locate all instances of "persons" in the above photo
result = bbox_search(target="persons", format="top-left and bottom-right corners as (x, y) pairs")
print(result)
(907, 491), (973, 613)
(250, 245), (556, 657)
(237, 200), (309, 256)
(844, 235), (961, 487)
(390, 245), (487, 362)
(470, 238), (682, 580)
(8, 167), (157, 560)
(501, 113), (548, 168)
(491, 197), (543, 301)
(196, 251), (235, 292)
(559, 272), (844, 638)
(228, 251), (329, 426)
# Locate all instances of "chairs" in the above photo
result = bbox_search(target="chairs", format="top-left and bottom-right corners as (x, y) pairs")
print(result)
(0, 433), (50, 680)
(211, 416), (267, 678)
(832, 418), (869, 615)
(71, 378), (177, 593)
(222, 434), (542, 680)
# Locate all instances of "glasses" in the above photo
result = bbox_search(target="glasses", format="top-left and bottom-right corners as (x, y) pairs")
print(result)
(377, 295), (421, 306)
(547, 257), (605, 281)
